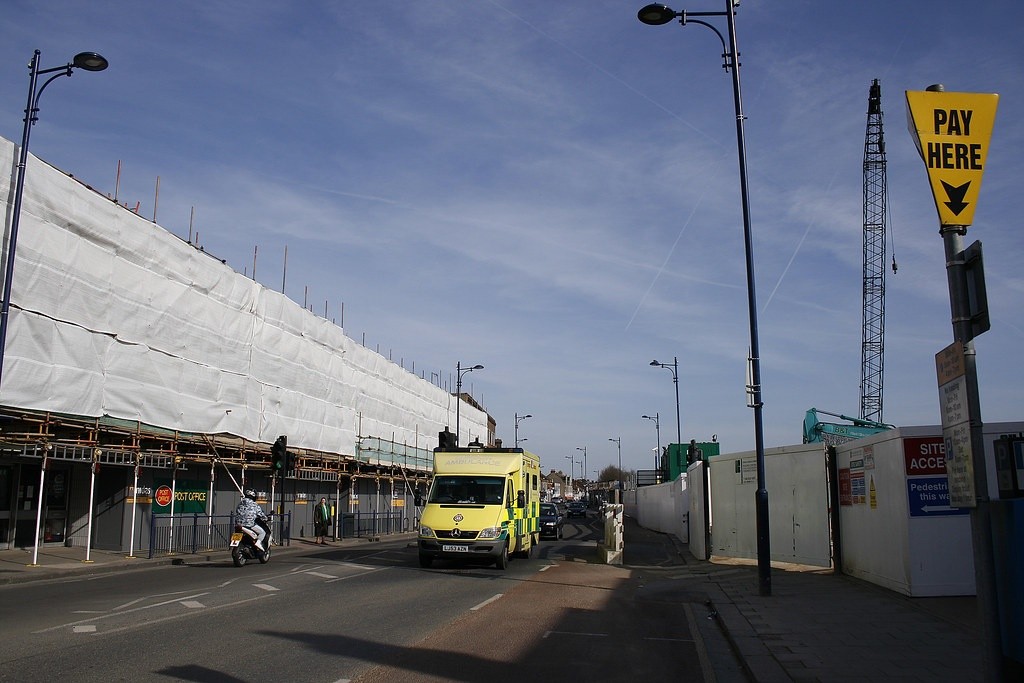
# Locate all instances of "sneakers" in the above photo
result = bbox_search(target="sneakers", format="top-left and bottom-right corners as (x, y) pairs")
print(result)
(255, 541), (265, 551)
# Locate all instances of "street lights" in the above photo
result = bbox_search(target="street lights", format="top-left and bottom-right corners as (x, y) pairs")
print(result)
(594, 470), (599, 477)
(515, 415), (532, 448)
(642, 413), (660, 473)
(637, 0), (771, 597)
(456, 365), (484, 449)
(609, 438), (621, 505)
(576, 461), (582, 478)
(576, 446), (586, 478)
(650, 357), (681, 474)
(565, 455), (573, 480)
(0, 52), (108, 383)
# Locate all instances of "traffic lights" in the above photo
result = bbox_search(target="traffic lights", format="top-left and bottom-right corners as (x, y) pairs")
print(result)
(272, 441), (285, 470)
(439, 433), (448, 449)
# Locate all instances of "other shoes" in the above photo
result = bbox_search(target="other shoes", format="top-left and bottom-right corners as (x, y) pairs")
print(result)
(316, 541), (320, 544)
(321, 542), (328, 545)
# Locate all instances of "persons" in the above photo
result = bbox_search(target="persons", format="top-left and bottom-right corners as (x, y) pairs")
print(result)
(440, 484), (454, 498)
(314, 497), (329, 545)
(688, 439), (700, 464)
(235, 487), (269, 552)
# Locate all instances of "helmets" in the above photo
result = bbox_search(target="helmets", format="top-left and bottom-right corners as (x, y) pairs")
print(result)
(245, 488), (257, 502)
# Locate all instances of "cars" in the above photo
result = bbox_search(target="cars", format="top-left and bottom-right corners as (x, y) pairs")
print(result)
(565, 501), (587, 518)
(552, 497), (561, 503)
(539, 503), (564, 540)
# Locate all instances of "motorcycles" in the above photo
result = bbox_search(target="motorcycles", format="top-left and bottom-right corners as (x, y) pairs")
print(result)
(230, 510), (275, 567)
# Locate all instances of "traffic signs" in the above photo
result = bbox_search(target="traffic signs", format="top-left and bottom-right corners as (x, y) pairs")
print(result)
(905, 89), (999, 234)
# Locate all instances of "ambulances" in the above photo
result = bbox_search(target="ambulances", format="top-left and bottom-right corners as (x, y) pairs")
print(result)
(417, 447), (541, 570)
